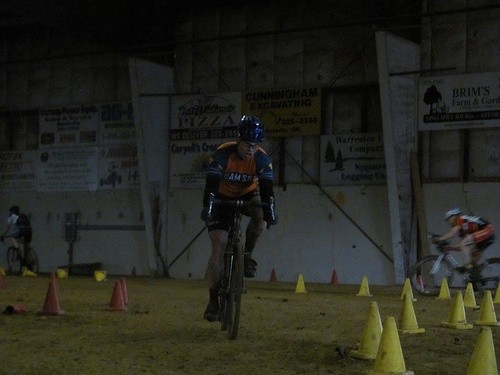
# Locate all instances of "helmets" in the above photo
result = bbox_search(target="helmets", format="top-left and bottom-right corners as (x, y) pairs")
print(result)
(238, 114), (265, 142)
(10, 207), (19, 213)
(444, 208), (460, 220)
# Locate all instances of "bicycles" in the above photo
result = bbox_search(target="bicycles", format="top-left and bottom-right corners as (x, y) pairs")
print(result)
(409, 232), (500, 299)
(202, 192), (275, 340)
(0, 232), (40, 278)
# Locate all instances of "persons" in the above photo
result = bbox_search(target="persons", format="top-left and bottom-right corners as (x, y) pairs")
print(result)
(432, 209), (495, 288)
(0, 206), (33, 269)
(200, 114), (279, 320)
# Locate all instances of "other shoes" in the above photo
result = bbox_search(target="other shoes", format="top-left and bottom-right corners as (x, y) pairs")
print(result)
(244, 258), (257, 277)
(204, 301), (218, 318)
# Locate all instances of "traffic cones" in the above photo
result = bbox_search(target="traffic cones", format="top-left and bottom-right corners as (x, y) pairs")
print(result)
(0, 266), (6, 278)
(38, 271), (65, 316)
(349, 301), (385, 360)
(292, 273), (309, 295)
(440, 291), (474, 331)
(267, 269), (278, 284)
(356, 273), (374, 297)
(434, 278), (453, 300)
(327, 269), (341, 287)
(465, 326), (499, 375)
(56, 268), (68, 280)
(94, 270), (106, 283)
(493, 280), (500, 303)
(396, 293), (425, 335)
(368, 316), (414, 375)
(398, 277), (418, 302)
(103, 276), (129, 313)
(473, 290), (500, 327)
(462, 282), (480, 310)
(21, 265), (38, 278)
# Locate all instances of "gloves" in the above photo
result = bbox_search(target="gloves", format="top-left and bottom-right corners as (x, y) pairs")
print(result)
(201, 207), (214, 222)
(263, 213), (279, 225)
(433, 237), (440, 243)
